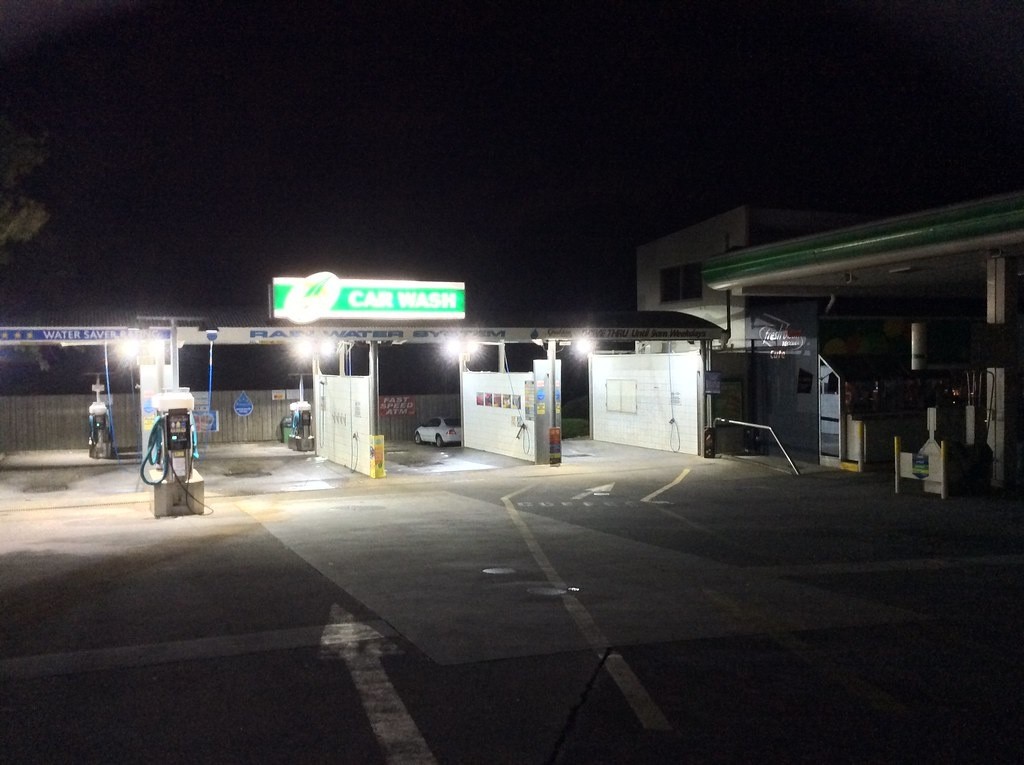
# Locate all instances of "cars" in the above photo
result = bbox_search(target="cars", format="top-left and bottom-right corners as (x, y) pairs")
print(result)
(413, 416), (461, 448)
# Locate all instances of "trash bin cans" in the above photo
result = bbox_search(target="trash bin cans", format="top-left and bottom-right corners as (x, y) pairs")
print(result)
(281, 418), (294, 444)
